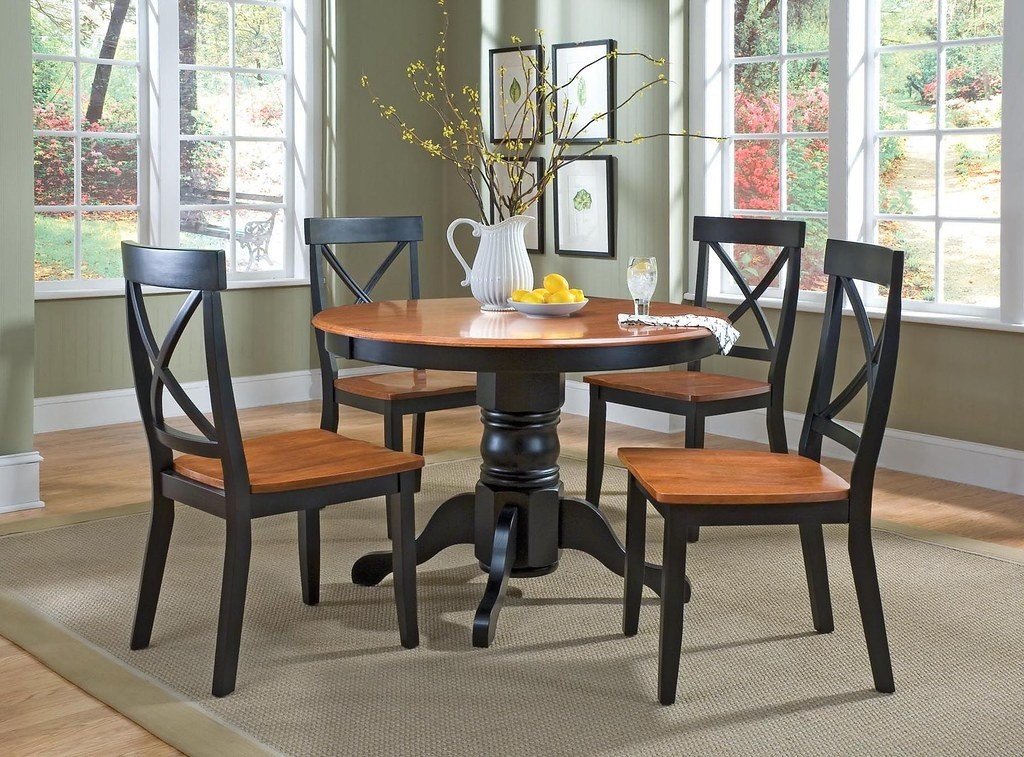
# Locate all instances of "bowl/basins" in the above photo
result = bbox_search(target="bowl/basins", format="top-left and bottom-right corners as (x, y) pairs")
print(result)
(507, 296), (589, 318)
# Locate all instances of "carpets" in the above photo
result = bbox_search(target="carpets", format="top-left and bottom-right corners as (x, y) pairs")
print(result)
(0, 444), (1024, 757)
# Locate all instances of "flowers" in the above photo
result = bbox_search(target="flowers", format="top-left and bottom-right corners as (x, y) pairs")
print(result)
(360, 0), (736, 227)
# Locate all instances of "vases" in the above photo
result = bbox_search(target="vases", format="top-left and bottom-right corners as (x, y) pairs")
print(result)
(446, 215), (537, 311)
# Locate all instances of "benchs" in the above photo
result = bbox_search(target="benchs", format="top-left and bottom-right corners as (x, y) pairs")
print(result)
(180, 183), (282, 272)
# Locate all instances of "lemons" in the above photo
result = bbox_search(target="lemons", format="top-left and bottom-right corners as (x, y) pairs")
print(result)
(637, 261), (647, 271)
(511, 273), (585, 304)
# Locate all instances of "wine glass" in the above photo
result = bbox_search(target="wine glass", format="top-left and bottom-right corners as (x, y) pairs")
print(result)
(626, 255), (658, 316)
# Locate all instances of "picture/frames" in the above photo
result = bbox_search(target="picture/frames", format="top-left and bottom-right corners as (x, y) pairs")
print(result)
(488, 44), (543, 143)
(490, 157), (543, 254)
(552, 39), (615, 145)
(553, 155), (616, 259)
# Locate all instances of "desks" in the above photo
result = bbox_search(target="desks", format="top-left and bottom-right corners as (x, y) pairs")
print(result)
(311, 296), (731, 648)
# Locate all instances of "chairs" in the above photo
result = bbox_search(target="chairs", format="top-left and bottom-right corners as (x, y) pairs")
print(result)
(583, 215), (808, 539)
(120, 237), (427, 699)
(303, 215), (481, 539)
(616, 239), (906, 708)
